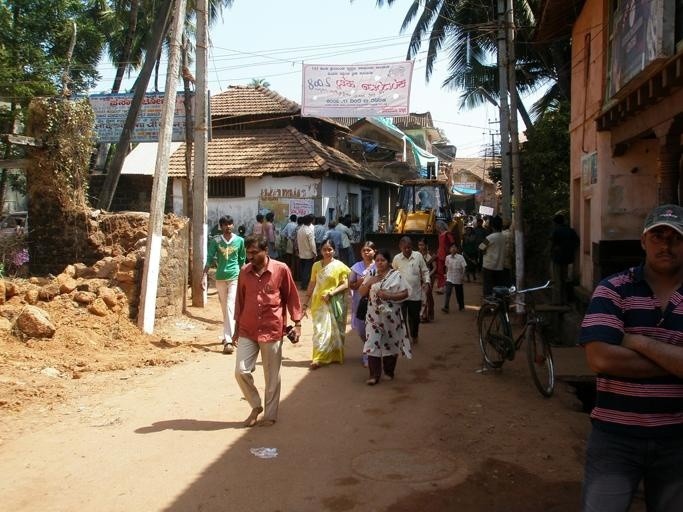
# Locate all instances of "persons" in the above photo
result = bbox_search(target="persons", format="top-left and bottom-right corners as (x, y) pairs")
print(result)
(414, 210), (516, 314)
(227, 233), (303, 429)
(13, 218), (24, 239)
(579, 204), (683, 511)
(543, 208), (579, 302)
(302, 235), (431, 384)
(234, 211), (364, 289)
(199, 216), (246, 354)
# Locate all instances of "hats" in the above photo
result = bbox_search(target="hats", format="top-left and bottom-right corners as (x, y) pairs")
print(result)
(641, 204), (683, 236)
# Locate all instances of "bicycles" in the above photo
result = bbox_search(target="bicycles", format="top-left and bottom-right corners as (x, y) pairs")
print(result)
(476, 279), (557, 397)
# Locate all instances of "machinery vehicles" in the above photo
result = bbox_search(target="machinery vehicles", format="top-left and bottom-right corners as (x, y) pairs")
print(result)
(365, 162), (465, 269)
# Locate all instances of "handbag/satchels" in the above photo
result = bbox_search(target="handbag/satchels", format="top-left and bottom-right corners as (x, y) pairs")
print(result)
(357, 293), (375, 320)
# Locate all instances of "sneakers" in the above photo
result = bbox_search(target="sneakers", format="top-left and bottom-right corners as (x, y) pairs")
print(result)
(367, 378), (380, 385)
(224, 344), (233, 354)
(244, 408), (275, 427)
